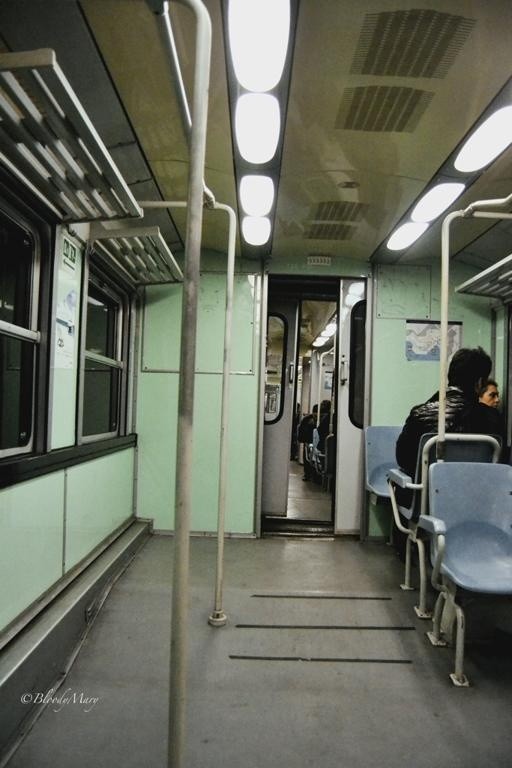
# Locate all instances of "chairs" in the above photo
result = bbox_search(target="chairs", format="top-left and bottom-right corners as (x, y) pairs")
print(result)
(418, 462), (511, 686)
(365, 425), (405, 546)
(321, 434), (334, 492)
(386, 432), (501, 620)
(313, 428), (325, 478)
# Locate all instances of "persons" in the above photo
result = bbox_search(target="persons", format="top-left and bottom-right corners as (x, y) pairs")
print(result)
(482, 381), (504, 415)
(396, 349), (511, 526)
(291, 397), (337, 493)
